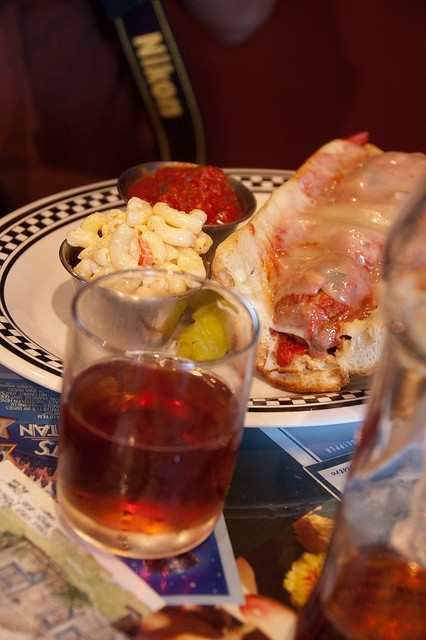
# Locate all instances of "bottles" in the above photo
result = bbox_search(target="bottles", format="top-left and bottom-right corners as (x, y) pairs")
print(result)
(293, 183), (425, 640)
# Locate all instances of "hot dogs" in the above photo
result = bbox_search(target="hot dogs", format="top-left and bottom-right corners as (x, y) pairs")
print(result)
(212, 131), (426, 398)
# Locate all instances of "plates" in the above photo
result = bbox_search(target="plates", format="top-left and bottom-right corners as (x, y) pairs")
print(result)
(0, 169), (372, 427)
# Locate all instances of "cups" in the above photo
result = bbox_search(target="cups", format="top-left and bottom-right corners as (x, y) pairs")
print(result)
(54, 267), (259, 560)
